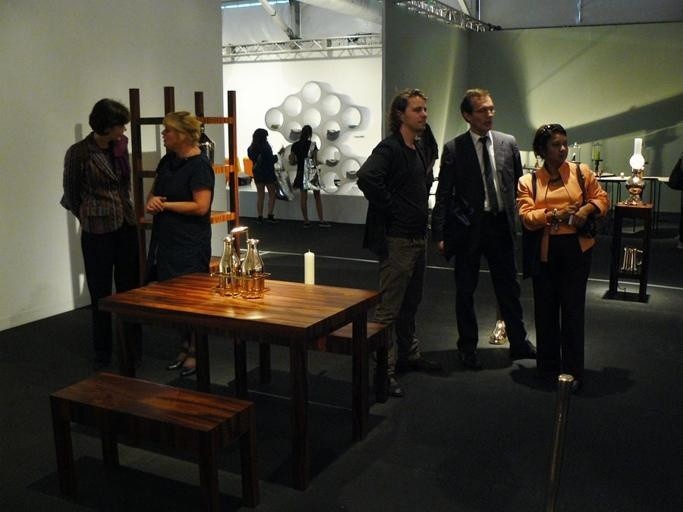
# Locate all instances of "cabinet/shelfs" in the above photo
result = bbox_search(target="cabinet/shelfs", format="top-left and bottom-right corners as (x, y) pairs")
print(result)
(609, 202), (653, 300)
(129, 87), (240, 284)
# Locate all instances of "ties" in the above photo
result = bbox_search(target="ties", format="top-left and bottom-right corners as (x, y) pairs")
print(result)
(478, 136), (498, 214)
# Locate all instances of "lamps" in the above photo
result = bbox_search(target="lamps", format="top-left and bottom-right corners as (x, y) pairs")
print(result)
(591, 141), (603, 177)
(624, 137), (646, 205)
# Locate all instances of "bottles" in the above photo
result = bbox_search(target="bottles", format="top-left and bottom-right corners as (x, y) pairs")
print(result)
(240, 238), (265, 299)
(219, 235), (242, 295)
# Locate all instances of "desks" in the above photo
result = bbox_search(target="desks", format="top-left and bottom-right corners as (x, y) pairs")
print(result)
(595, 172), (615, 212)
(596, 176), (669, 235)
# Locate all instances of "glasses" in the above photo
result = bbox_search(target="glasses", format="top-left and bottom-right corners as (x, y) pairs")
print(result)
(474, 109), (496, 115)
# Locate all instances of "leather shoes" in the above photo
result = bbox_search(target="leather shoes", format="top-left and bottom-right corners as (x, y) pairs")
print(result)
(375, 376), (405, 397)
(167, 352), (197, 375)
(457, 349), (480, 364)
(514, 347), (536, 359)
(395, 353), (441, 371)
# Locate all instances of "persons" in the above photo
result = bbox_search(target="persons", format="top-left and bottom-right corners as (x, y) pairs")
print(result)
(356, 89), (443, 396)
(59, 98), (143, 377)
(417, 123), (438, 198)
(289, 125), (333, 228)
(248, 129), (278, 224)
(431, 88), (537, 369)
(516, 124), (610, 395)
(143, 111), (215, 376)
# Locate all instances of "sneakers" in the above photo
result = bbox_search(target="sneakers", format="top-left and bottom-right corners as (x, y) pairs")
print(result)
(489, 319), (508, 344)
(255, 214), (279, 225)
(303, 221), (331, 228)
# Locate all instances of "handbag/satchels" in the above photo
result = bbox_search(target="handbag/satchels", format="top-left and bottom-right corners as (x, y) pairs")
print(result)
(573, 205), (595, 238)
(273, 169), (295, 200)
(303, 157), (323, 191)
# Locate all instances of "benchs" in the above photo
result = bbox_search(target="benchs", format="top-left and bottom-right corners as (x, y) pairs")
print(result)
(51, 371), (259, 510)
(248, 321), (389, 416)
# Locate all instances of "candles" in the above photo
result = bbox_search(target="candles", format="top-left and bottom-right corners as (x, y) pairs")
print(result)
(304, 248), (315, 285)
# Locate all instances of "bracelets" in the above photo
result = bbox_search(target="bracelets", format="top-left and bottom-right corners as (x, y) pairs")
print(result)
(552, 208), (557, 222)
